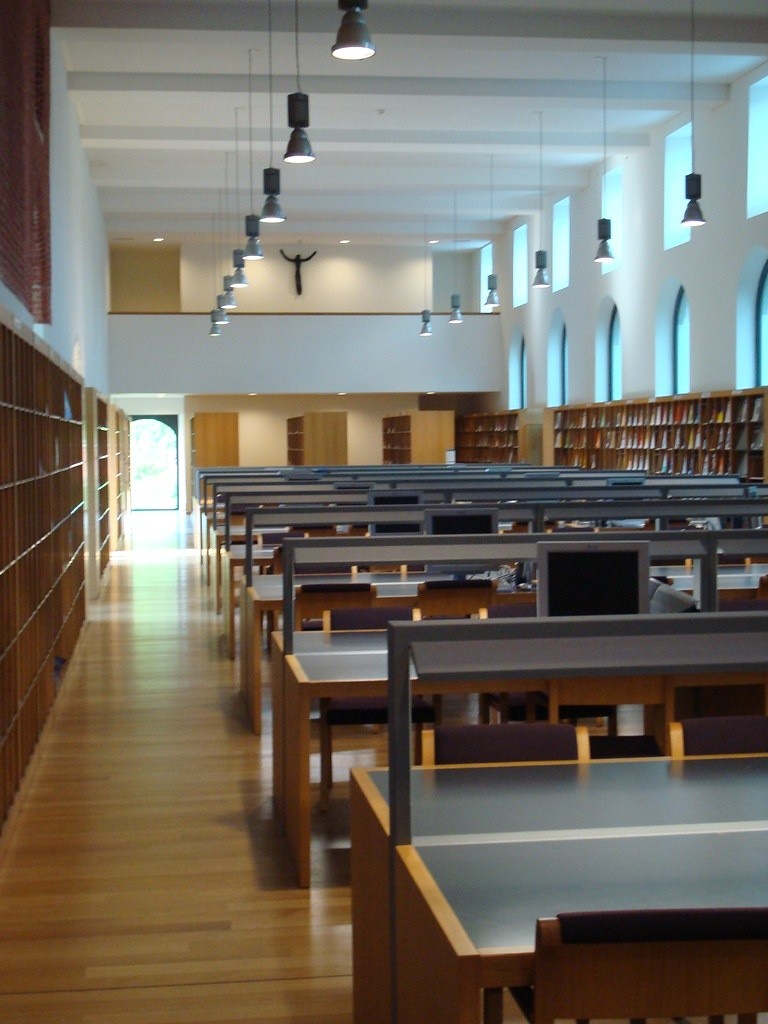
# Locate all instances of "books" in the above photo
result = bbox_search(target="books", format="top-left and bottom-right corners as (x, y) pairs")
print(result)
(456, 413), (517, 462)
(552, 395), (766, 475)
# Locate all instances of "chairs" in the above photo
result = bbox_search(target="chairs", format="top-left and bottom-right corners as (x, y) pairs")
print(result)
(295, 581), (377, 635)
(322, 608), (435, 792)
(270, 546), (360, 650)
(482, 909), (768, 1024)
(416, 723), (593, 766)
(257, 531), (308, 626)
(479, 604), (619, 734)
(414, 576), (497, 621)
(668, 717), (768, 757)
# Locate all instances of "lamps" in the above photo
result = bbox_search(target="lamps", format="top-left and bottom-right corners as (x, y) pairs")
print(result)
(447, 185), (465, 327)
(680, 0), (708, 229)
(208, 0), (377, 337)
(481, 152), (501, 309)
(591, 54), (618, 265)
(531, 110), (554, 292)
(417, 217), (435, 341)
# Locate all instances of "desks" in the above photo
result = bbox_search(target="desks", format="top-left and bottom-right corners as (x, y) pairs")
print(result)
(194, 465), (768, 1023)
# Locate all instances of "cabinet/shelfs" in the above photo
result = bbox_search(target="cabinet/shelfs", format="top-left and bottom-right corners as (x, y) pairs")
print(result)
(284, 411), (347, 467)
(383, 410), (455, 467)
(188, 411), (240, 513)
(0, 307), (132, 832)
(461, 411), (532, 463)
(544, 385), (768, 475)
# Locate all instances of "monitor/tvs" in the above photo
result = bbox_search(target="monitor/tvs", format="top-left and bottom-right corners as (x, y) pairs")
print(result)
(525, 473), (645, 486)
(276, 470), (501, 579)
(535, 542), (651, 618)
